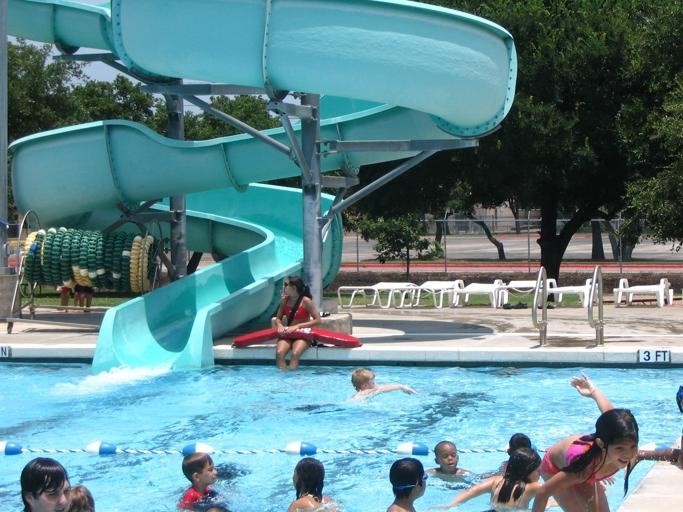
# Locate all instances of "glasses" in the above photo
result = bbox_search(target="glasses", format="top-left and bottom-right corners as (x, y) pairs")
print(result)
(284, 282), (289, 288)
(421, 473), (427, 481)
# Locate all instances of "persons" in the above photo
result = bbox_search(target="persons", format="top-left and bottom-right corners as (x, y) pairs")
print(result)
(531, 371), (639, 512)
(21, 457), (71, 512)
(73, 285), (94, 312)
(67, 485), (94, 512)
(349, 368), (416, 400)
(447, 448), (541, 512)
(424, 441), (471, 475)
(507, 433), (531, 455)
(176, 452), (217, 509)
(386, 458), (428, 512)
(287, 458), (332, 512)
(275, 275), (320, 369)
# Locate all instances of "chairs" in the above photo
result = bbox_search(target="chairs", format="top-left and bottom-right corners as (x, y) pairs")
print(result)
(392, 279), (464, 309)
(458, 279), (506, 308)
(338, 282), (421, 309)
(546, 278), (599, 308)
(495, 280), (543, 308)
(613, 278), (673, 308)
